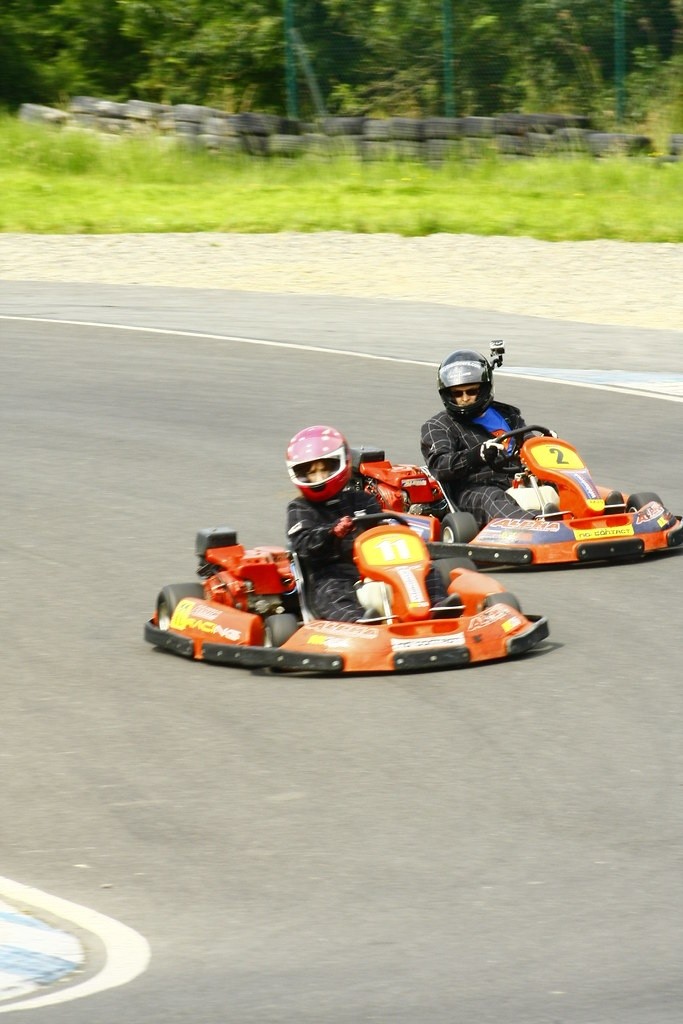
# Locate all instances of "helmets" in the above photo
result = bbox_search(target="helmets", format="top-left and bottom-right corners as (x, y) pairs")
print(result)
(285, 425), (352, 503)
(436, 350), (493, 422)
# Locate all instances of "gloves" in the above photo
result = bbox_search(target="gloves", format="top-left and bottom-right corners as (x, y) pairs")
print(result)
(327, 516), (356, 539)
(467, 437), (504, 474)
(542, 428), (558, 439)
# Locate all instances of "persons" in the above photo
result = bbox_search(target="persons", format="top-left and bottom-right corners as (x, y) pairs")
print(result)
(421, 351), (625, 531)
(286, 425), (463, 624)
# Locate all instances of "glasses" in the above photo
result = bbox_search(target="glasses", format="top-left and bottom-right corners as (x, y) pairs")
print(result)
(450, 385), (481, 397)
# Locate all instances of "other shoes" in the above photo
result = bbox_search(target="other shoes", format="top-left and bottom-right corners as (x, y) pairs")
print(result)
(432, 593), (463, 619)
(362, 609), (383, 625)
(604, 491), (624, 513)
(544, 503), (563, 521)
